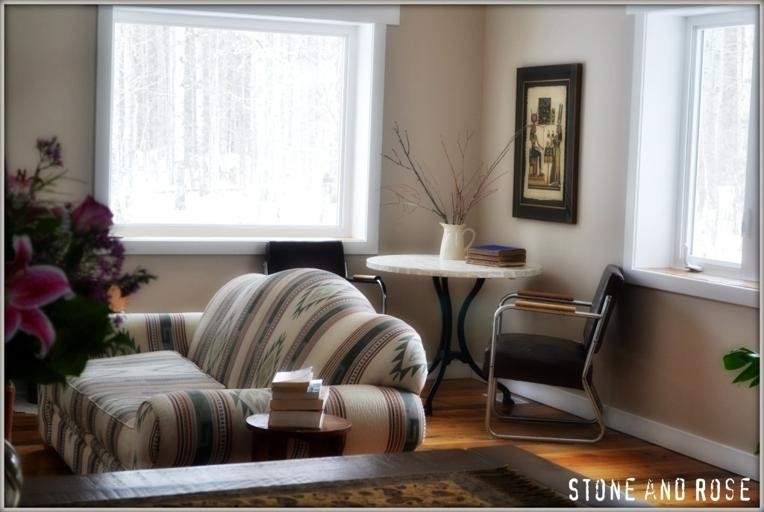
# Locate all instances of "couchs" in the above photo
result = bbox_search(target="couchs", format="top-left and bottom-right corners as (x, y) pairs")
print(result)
(37, 266), (429, 474)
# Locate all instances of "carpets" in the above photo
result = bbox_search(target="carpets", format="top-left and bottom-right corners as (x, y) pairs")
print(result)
(24, 464), (578, 507)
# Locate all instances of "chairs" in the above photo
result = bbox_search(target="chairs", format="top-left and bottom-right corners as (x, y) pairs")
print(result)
(264, 240), (387, 317)
(480, 264), (625, 444)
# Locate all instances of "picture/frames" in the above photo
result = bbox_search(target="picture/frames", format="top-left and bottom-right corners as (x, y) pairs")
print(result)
(511, 63), (582, 226)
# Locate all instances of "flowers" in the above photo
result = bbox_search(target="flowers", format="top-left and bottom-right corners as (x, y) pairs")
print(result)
(4, 134), (157, 391)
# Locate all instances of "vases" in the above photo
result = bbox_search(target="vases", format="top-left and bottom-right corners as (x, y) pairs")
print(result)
(4, 433), (25, 509)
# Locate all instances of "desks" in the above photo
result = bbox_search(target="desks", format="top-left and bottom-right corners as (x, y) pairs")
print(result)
(244, 411), (352, 461)
(23, 440), (655, 506)
(365, 255), (543, 418)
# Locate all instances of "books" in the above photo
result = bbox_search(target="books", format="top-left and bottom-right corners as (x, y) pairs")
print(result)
(269, 366), (331, 429)
(466, 245), (526, 269)
